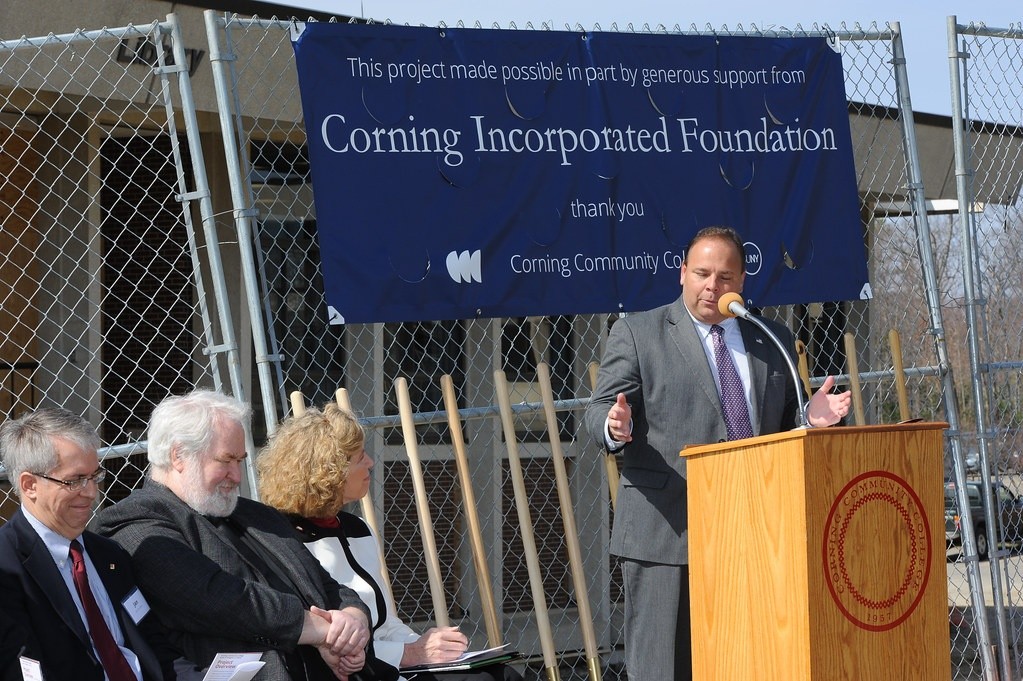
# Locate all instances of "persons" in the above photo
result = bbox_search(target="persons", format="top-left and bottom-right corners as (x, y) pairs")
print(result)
(258, 404), (521, 681)
(585, 227), (852, 681)
(0, 411), (208, 681)
(96, 391), (400, 681)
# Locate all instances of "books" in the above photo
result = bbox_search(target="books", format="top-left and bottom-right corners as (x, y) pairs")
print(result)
(399, 651), (525, 672)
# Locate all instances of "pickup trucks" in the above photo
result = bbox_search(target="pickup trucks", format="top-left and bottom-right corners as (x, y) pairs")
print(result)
(943, 481), (1023, 562)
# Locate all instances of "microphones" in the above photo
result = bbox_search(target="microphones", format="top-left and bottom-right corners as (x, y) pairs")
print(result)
(718, 291), (812, 432)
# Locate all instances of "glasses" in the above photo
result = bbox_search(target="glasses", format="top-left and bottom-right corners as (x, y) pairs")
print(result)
(32, 465), (108, 491)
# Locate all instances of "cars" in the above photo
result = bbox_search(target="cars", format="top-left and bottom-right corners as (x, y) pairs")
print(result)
(965, 440), (1007, 475)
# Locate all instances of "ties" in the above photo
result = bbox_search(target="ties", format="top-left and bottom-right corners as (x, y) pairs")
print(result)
(709, 324), (754, 441)
(68, 539), (138, 681)
